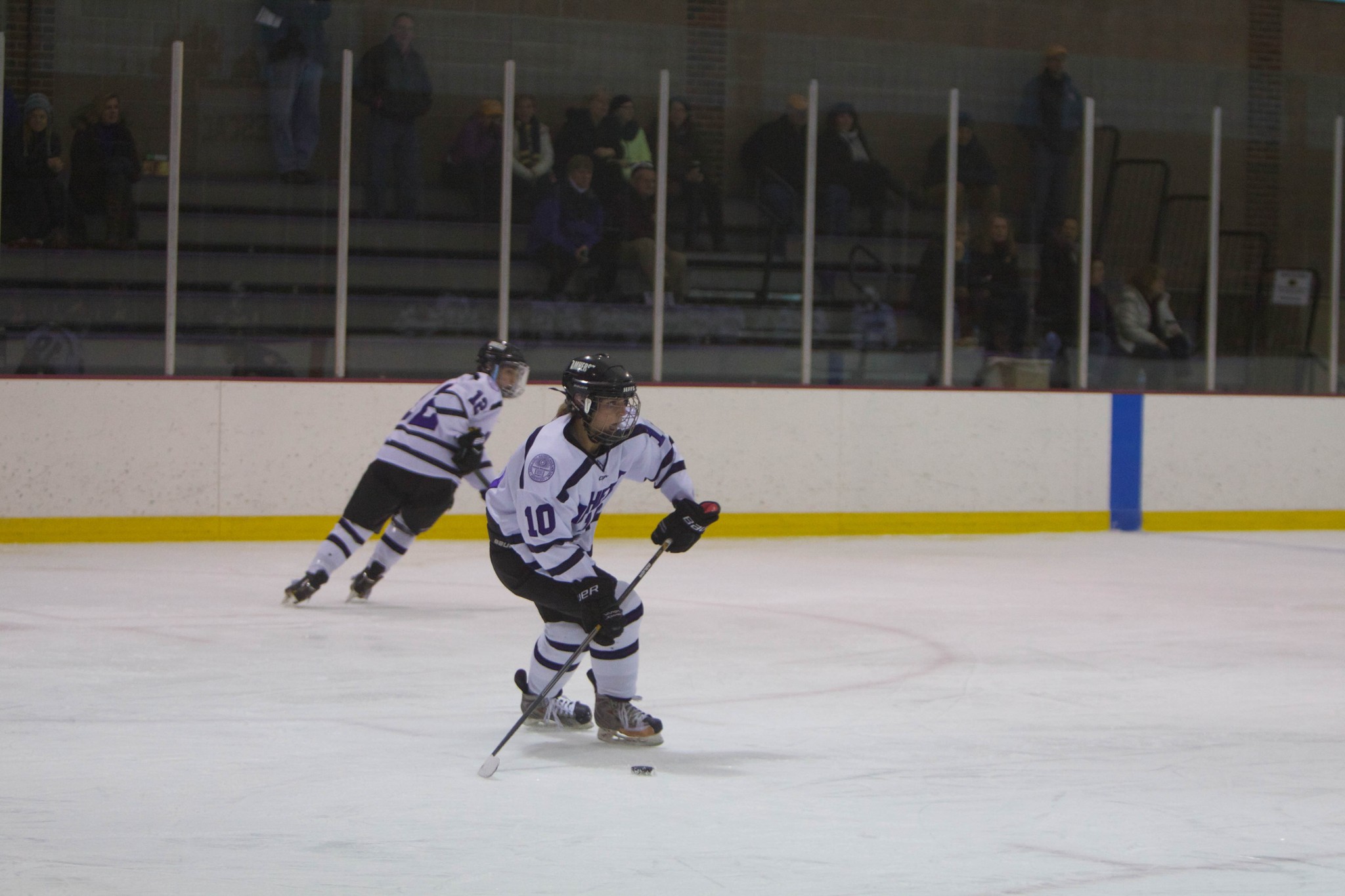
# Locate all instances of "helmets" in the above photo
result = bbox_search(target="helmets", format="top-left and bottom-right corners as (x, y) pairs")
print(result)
(549, 353), (642, 446)
(478, 338), (529, 399)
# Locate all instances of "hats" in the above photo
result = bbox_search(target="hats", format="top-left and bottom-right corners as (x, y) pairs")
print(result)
(480, 98), (503, 118)
(23, 92), (53, 126)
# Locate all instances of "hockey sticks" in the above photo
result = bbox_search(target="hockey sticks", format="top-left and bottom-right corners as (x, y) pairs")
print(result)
(473, 467), (490, 488)
(477, 536), (674, 780)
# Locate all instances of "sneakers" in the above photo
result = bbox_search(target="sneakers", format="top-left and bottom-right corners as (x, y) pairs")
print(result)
(343, 560), (386, 603)
(284, 569), (330, 604)
(585, 669), (664, 746)
(514, 669), (594, 730)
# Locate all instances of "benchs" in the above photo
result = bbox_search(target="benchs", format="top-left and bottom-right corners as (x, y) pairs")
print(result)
(0, 176), (1058, 388)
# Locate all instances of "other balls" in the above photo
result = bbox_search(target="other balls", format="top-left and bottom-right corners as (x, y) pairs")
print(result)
(631, 765), (655, 776)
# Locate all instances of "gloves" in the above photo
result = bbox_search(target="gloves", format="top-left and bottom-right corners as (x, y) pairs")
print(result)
(452, 428), (492, 475)
(651, 499), (720, 553)
(572, 565), (624, 647)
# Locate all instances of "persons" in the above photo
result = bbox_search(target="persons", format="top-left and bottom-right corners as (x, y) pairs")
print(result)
(284, 339), (530, 606)
(260, 1), (331, 184)
(485, 355), (720, 747)
(440, 96), (726, 303)
(734, 44), (1191, 391)
(2, 93), (69, 247)
(352, 13), (432, 221)
(66, 94), (141, 250)
